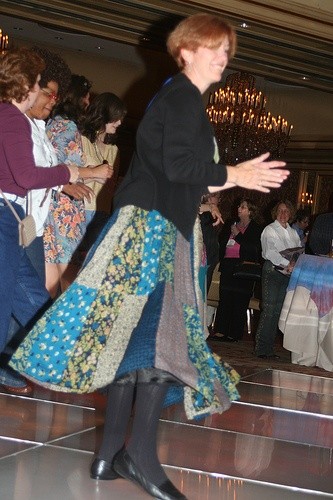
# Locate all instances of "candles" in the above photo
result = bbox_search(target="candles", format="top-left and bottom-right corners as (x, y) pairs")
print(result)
(205, 71), (293, 140)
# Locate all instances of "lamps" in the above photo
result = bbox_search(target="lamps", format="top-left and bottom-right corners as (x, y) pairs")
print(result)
(0, 28), (9, 54)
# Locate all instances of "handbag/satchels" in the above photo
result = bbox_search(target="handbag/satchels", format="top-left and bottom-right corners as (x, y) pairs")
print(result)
(18, 214), (37, 247)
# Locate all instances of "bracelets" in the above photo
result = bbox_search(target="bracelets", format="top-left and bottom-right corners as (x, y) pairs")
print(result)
(209, 203), (212, 212)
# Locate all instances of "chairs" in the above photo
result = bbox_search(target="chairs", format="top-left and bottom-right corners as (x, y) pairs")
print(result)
(207, 263), (260, 335)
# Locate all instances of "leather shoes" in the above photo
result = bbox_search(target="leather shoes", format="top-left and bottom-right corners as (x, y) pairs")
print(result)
(90, 456), (122, 480)
(112, 447), (188, 500)
(0, 384), (33, 396)
(257, 353), (280, 359)
(205, 333), (236, 342)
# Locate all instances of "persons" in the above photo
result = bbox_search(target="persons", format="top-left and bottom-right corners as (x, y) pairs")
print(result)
(253, 199), (304, 361)
(90, 13), (291, 500)
(291, 210), (309, 247)
(44, 74), (116, 299)
(6, 69), (96, 354)
(198, 191), (225, 339)
(0, 46), (79, 388)
(77, 92), (128, 262)
(211, 198), (266, 342)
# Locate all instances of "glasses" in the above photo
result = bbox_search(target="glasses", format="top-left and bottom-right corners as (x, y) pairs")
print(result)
(40, 87), (59, 103)
(237, 205), (248, 209)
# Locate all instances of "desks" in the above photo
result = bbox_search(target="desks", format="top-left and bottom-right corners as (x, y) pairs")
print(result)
(278, 254), (333, 371)
